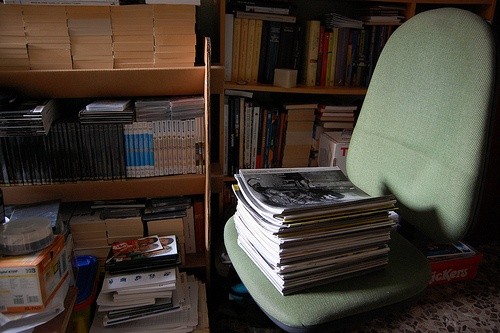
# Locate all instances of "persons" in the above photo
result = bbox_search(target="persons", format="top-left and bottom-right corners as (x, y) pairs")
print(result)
(159, 238), (174, 246)
(111, 237), (158, 248)
(113, 243), (159, 255)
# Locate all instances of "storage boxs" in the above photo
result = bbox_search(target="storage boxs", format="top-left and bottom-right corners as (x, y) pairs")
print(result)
(0, 235), (69, 315)
(71, 255), (100, 333)
(317, 132), (351, 176)
(426, 239), (482, 283)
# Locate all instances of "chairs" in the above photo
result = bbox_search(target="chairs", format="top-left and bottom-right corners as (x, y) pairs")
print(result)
(223, 9), (496, 333)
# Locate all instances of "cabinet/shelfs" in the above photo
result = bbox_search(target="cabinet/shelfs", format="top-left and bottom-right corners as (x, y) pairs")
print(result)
(0, 0), (496, 285)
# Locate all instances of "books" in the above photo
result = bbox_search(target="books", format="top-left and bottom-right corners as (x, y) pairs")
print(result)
(0, 189), (210, 333)
(223, 89), (358, 177)
(0, 0), (201, 72)
(231, 166), (400, 296)
(0, 93), (206, 187)
(225, 1), (407, 90)
